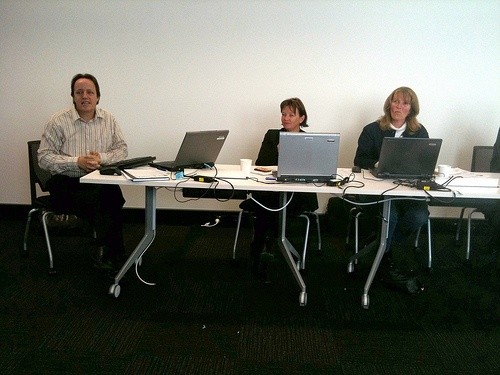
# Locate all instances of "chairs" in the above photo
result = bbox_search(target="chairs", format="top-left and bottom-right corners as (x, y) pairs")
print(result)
(231, 201), (321, 274)
(344, 207), (433, 276)
(22, 139), (104, 277)
(454, 145), (494, 266)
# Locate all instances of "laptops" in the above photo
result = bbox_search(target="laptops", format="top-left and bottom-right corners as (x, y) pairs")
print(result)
(149, 129), (229, 172)
(272, 131), (340, 183)
(369, 137), (442, 179)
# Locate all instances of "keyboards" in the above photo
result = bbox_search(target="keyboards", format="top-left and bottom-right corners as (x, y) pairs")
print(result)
(103, 156), (156, 169)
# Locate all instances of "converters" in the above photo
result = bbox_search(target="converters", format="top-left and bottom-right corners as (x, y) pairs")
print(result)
(417, 179), (437, 190)
(328, 179), (344, 186)
(194, 175), (213, 183)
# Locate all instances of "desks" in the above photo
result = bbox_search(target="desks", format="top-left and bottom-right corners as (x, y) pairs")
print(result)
(79, 164), (500, 311)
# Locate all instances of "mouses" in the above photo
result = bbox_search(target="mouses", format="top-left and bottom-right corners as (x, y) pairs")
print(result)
(352, 165), (361, 173)
(100, 166), (121, 175)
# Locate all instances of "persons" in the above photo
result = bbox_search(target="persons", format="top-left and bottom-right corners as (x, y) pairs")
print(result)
(248, 97), (319, 275)
(36, 73), (134, 286)
(353, 86), (430, 279)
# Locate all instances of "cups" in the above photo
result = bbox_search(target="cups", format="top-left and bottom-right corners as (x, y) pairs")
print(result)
(438, 165), (451, 178)
(240, 159), (252, 175)
